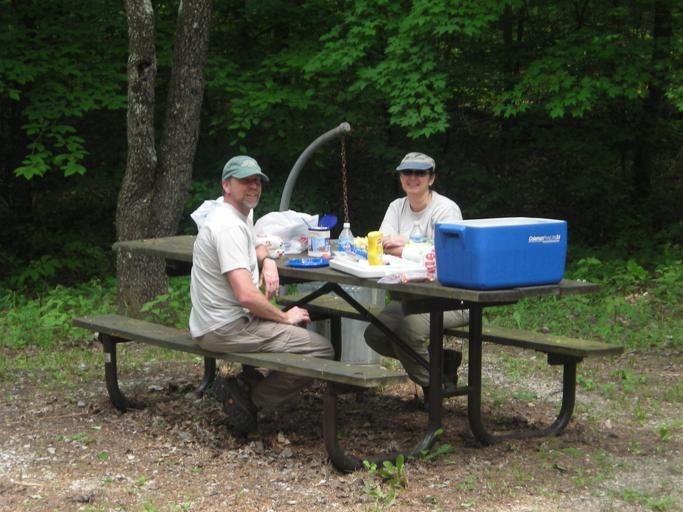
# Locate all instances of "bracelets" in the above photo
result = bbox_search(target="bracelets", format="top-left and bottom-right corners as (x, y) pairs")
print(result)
(260, 252), (276, 265)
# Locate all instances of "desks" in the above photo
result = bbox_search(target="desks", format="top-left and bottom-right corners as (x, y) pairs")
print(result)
(110, 235), (600, 474)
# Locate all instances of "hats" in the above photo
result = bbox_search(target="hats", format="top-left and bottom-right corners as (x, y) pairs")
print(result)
(222, 154), (269, 188)
(396, 151), (437, 173)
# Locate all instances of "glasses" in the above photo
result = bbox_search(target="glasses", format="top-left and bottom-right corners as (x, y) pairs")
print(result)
(402, 169), (433, 177)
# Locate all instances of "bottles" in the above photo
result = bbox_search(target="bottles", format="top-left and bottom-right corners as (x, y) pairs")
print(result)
(337, 223), (354, 251)
(408, 222), (424, 243)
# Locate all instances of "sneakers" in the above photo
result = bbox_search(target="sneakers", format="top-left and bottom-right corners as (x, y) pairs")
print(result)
(442, 348), (464, 392)
(420, 384), (442, 410)
(229, 391), (257, 441)
(213, 377), (260, 431)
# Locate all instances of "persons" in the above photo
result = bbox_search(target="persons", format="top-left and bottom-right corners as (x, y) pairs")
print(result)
(187, 154), (337, 441)
(361, 152), (470, 409)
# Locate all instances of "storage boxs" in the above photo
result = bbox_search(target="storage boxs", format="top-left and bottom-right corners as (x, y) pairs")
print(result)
(434, 217), (569, 290)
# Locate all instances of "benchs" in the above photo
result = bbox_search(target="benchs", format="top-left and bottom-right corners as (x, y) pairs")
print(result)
(276, 292), (624, 436)
(73, 313), (407, 474)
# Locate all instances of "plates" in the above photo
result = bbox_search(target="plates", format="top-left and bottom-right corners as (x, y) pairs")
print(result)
(286, 257), (330, 268)
